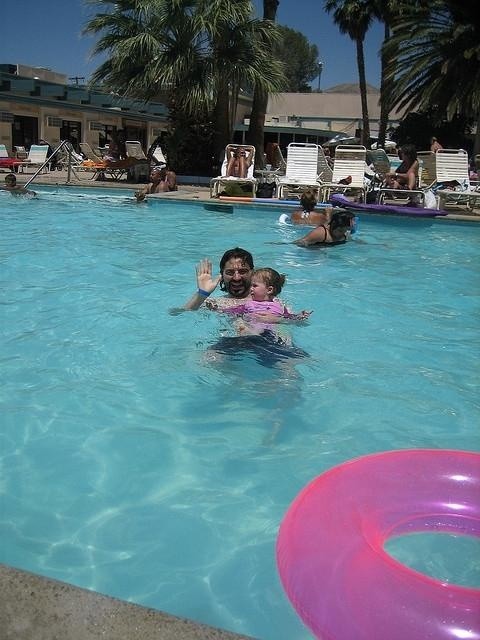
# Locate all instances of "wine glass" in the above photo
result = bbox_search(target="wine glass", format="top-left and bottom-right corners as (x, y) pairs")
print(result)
(266, 164), (271, 171)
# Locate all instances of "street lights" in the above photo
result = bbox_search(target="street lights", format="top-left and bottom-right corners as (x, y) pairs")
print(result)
(318, 62), (323, 91)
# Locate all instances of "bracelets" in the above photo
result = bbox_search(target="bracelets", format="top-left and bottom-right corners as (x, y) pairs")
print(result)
(196, 288), (210, 297)
(396, 173), (399, 177)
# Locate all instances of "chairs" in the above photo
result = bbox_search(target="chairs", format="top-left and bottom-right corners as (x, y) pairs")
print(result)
(211, 142), (479, 209)
(0, 141), (169, 182)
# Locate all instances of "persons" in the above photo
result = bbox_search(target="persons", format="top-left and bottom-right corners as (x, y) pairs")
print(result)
(265, 206), (357, 251)
(0, 174), (38, 200)
(221, 145), (255, 178)
(161, 169), (178, 191)
(134, 168), (169, 200)
(290, 191), (335, 226)
(185, 245), (314, 444)
(384, 143), (419, 190)
(430, 136), (444, 155)
(204, 267), (315, 337)
(108, 129), (140, 169)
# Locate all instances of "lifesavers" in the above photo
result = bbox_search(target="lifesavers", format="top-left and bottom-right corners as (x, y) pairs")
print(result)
(276, 447), (479, 640)
